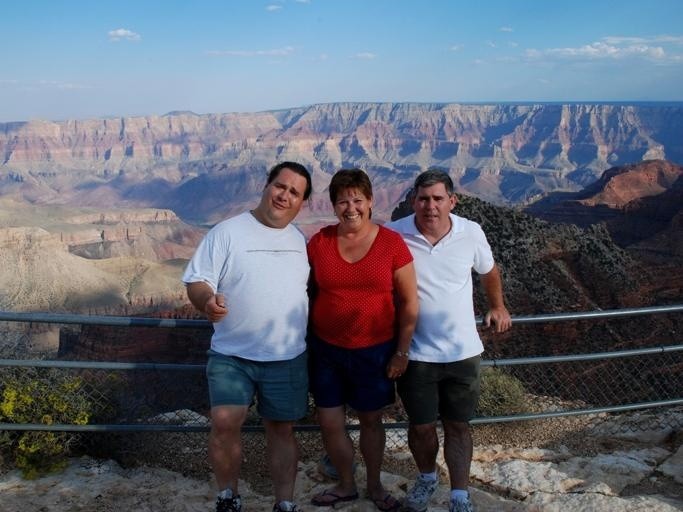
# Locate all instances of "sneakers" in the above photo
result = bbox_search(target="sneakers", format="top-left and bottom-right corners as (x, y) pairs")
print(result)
(403, 476), (439, 512)
(216, 489), (241, 512)
(273, 501), (304, 512)
(450, 492), (473, 512)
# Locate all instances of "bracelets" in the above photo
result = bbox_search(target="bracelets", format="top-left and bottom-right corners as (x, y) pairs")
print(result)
(397, 351), (409, 357)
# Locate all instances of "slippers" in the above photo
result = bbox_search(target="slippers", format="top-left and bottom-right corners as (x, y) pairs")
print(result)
(369, 492), (400, 512)
(311, 490), (358, 506)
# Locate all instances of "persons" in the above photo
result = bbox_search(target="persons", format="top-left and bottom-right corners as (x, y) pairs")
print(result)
(304, 169), (419, 512)
(181, 162), (312, 512)
(385, 170), (513, 512)
